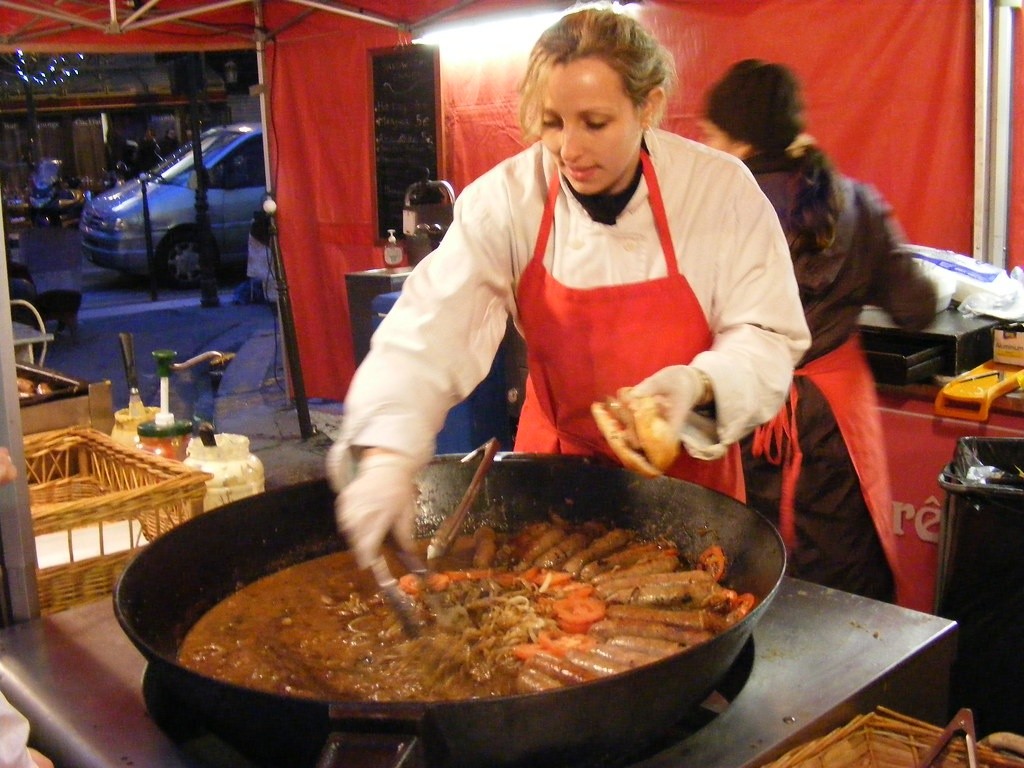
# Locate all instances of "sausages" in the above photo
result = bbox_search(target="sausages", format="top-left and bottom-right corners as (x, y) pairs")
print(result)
(470, 517), (729, 695)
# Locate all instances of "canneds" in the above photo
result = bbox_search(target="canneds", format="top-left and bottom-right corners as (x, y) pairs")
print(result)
(107, 403), (264, 517)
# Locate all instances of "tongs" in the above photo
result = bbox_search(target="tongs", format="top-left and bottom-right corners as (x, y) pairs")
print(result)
(427, 435), (499, 564)
(916, 707), (980, 768)
(327, 442), (457, 640)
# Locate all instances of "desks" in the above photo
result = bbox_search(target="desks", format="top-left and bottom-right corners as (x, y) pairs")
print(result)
(344, 265), (415, 367)
(11, 321), (54, 365)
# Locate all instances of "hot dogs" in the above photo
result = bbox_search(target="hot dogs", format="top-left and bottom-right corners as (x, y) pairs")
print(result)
(591, 384), (680, 480)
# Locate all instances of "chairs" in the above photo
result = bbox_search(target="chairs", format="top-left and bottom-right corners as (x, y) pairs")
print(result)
(9, 280), (82, 355)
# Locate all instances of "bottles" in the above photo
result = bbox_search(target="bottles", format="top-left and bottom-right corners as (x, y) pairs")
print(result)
(383, 229), (403, 268)
(180, 433), (266, 513)
(133, 420), (194, 462)
(109, 406), (160, 450)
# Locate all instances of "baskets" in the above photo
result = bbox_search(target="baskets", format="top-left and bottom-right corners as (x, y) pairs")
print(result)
(761, 704), (1018, 768)
(22, 423), (214, 614)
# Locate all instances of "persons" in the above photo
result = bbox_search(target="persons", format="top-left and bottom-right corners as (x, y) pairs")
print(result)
(324, 8), (814, 570)
(694, 58), (936, 716)
(98, 125), (160, 180)
(160, 126), (180, 156)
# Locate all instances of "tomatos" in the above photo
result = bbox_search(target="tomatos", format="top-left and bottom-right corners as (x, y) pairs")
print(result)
(398, 565), (607, 657)
(730, 591), (753, 623)
(695, 545), (725, 581)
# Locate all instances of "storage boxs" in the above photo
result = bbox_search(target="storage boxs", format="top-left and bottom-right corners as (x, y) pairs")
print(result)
(994, 328), (1024, 367)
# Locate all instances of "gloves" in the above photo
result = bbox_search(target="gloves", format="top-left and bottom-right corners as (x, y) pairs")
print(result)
(336, 455), (423, 569)
(629, 364), (705, 432)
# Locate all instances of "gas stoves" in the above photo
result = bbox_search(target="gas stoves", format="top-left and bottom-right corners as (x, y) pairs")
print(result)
(1, 573), (962, 768)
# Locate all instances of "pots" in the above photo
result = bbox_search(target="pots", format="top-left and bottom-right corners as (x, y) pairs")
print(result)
(113, 453), (788, 768)
